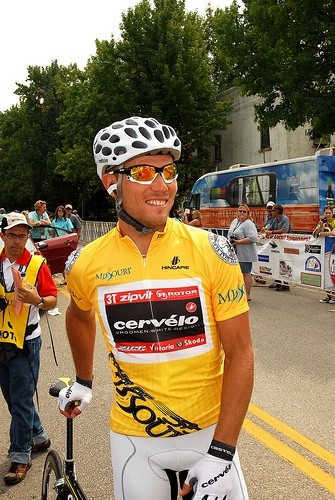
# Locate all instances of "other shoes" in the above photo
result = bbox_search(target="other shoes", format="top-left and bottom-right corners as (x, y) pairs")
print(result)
(319, 295), (331, 303)
(60, 280), (66, 285)
(277, 285), (290, 291)
(328, 296), (335, 304)
(269, 283), (277, 288)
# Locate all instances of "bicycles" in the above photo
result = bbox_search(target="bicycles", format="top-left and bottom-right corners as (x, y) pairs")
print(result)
(41, 377), (89, 500)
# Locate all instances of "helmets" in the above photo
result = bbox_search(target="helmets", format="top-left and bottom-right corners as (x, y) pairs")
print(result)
(93, 118), (181, 180)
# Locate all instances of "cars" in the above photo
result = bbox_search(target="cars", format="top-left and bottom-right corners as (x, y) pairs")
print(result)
(0, 225), (78, 275)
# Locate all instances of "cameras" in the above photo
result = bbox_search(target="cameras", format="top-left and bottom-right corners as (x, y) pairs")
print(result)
(229, 235), (238, 248)
(321, 217), (326, 223)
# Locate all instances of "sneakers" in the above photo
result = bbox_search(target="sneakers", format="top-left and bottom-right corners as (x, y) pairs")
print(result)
(31, 438), (50, 451)
(4, 459), (32, 484)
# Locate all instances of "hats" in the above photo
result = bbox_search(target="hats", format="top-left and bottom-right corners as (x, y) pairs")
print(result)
(266, 201), (275, 207)
(65, 204), (72, 210)
(1, 212), (33, 230)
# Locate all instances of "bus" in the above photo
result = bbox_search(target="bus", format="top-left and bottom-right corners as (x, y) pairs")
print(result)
(184, 147), (335, 233)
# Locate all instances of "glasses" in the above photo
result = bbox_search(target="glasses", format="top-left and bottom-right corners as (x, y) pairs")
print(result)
(108, 163), (179, 186)
(3, 233), (27, 240)
(58, 210), (64, 213)
(238, 210), (247, 214)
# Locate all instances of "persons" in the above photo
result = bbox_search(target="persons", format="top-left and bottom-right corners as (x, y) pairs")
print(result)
(0, 200), (335, 484)
(57, 117), (255, 500)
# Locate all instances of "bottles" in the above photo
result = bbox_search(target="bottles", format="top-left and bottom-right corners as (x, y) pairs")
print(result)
(326, 184), (334, 216)
(20, 272), (33, 306)
